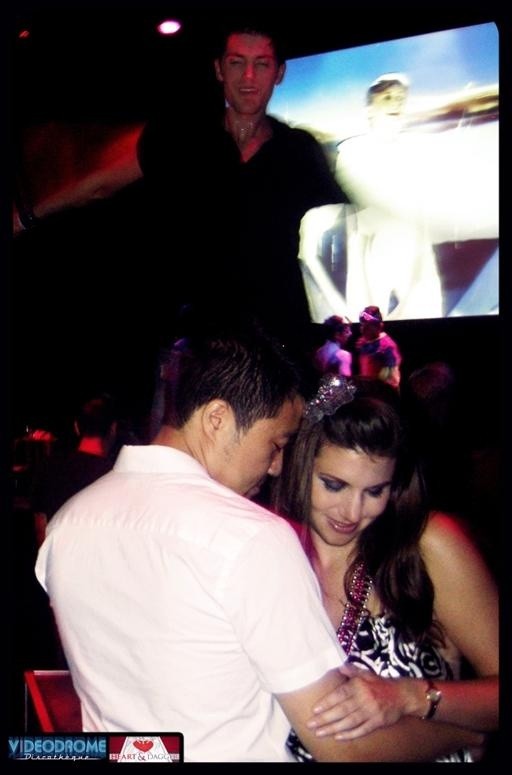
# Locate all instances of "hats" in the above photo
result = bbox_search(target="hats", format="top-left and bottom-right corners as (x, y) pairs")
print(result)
(359, 306), (382, 323)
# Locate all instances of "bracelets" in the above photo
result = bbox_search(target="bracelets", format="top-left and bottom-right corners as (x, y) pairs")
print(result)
(18, 207), (38, 228)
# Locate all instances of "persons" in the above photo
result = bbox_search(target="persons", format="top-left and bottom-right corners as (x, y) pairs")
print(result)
(11, 13), (343, 306)
(0, 306), (500, 763)
(337, 73), (444, 319)
